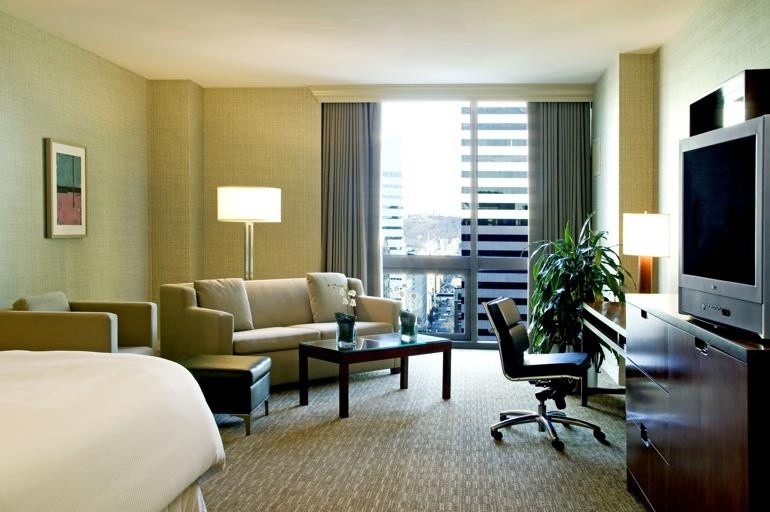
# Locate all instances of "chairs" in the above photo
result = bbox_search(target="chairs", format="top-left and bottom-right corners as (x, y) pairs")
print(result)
(0, 302), (159, 356)
(481, 295), (606, 451)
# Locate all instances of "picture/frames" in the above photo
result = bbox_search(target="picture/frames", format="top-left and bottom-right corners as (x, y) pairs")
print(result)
(46, 137), (87, 239)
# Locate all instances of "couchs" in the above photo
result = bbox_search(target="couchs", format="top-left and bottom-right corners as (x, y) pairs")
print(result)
(159, 276), (402, 386)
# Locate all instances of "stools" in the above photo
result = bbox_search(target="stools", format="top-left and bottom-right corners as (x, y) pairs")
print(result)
(178, 353), (272, 436)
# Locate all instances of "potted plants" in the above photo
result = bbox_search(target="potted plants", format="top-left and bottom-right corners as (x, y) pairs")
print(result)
(520, 210), (636, 394)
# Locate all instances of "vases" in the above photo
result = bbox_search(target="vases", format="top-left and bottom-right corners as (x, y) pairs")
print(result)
(334, 317), (355, 352)
(400, 311), (417, 345)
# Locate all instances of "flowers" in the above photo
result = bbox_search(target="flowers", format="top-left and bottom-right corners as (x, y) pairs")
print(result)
(327, 282), (359, 343)
(387, 282), (420, 335)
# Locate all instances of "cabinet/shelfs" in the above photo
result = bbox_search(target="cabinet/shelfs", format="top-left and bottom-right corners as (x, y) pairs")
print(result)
(580, 293), (770, 512)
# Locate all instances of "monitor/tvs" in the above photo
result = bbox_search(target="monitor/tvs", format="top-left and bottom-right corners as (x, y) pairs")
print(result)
(678, 115), (770, 340)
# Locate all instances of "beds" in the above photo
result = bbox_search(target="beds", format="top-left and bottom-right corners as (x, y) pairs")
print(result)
(0, 349), (228, 512)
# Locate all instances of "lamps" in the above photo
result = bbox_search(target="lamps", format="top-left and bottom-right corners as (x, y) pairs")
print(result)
(216, 186), (282, 280)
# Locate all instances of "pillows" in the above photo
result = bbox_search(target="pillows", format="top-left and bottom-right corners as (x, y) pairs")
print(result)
(193, 277), (254, 332)
(306, 271), (356, 323)
(12, 290), (71, 312)
